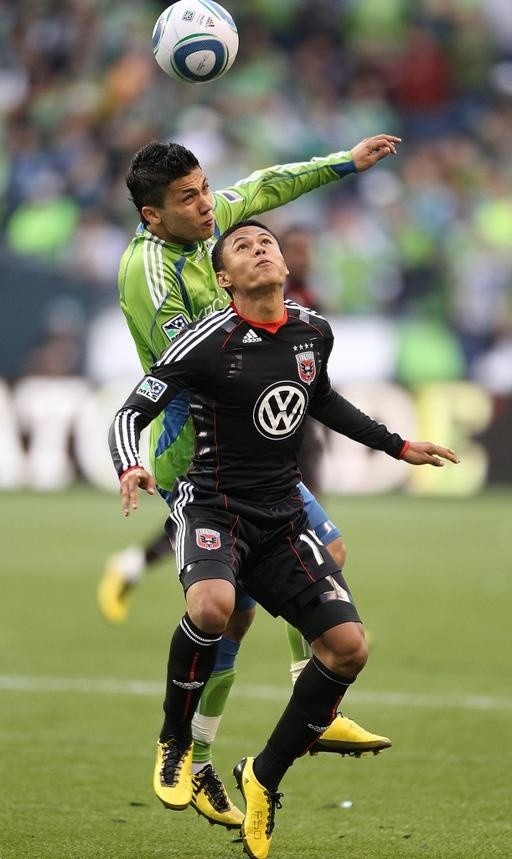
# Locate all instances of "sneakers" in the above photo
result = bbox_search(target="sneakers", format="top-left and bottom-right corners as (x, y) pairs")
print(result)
(232, 756), (278, 859)
(308, 713), (393, 758)
(152, 737), (196, 812)
(96, 551), (129, 627)
(188, 763), (246, 830)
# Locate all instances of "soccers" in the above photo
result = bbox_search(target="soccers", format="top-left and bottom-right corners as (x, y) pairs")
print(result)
(153, 0), (238, 84)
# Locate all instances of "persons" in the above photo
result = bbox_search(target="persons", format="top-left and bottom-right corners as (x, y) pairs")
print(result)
(108, 216), (459, 859)
(99, 520), (180, 630)
(277, 223), (328, 506)
(114, 134), (408, 831)
(0, 0), (512, 395)
